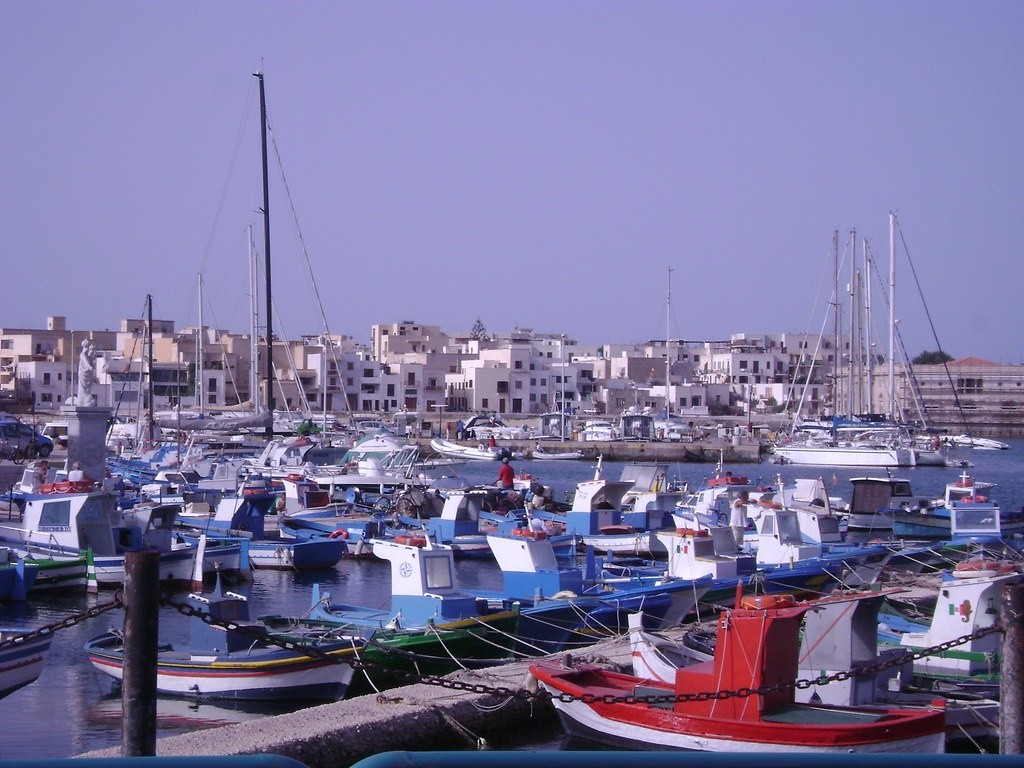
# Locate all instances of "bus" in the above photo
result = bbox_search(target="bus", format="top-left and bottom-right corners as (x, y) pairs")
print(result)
(310, 414), (336, 428)
(350, 413), (379, 427)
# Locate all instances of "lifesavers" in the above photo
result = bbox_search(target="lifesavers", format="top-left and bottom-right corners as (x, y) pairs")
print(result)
(288, 474), (304, 481)
(395, 535), (426, 547)
(512, 528), (547, 539)
(676, 528), (708, 537)
(741, 594), (795, 609)
(956, 480), (973, 487)
(962, 495), (988, 503)
(955, 561), (999, 570)
(760, 500), (782, 509)
(243, 489), (267, 495)
(329, 529), (348, 539)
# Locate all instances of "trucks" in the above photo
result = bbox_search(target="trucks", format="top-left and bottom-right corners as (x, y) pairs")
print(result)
(299, 419), (327, 436)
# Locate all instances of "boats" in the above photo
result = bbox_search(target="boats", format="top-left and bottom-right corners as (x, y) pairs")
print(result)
(0, 439), (1024, 768)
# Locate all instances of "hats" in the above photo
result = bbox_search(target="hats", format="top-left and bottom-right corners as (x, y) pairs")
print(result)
(503, 457), (508, 462)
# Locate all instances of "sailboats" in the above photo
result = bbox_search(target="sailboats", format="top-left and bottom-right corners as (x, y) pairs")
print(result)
(19, 213), (1009, 466)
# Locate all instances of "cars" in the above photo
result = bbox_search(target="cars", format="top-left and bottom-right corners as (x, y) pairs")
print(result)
(0, 416), (54, 454)
(41, 422), (68, 440)
(466, 421), (513, 440)
(104, 415), (163, 452)
(358, 421), (395, 436)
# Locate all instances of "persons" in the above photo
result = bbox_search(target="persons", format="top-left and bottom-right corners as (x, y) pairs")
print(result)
(733, 424), (740, 436)
(445, 411), (544, 453)
(730, 490), (757, 553)
(498, 458), (515, 490)
